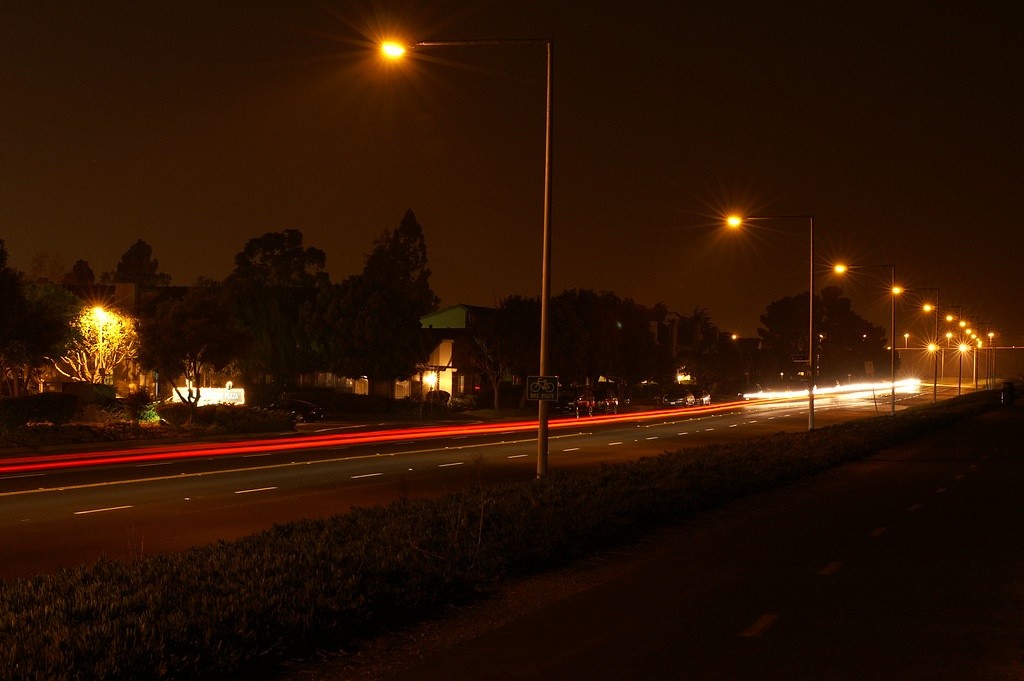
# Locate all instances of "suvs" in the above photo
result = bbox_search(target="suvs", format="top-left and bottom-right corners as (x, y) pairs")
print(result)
(590, 386), (619, 417)
(547, 385), (596, 419)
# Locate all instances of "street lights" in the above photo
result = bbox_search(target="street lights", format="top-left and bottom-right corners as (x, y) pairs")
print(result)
(892, 286), (939, 407)
(375, 35), (555, 482)
(835, 263), (895, 417)
(726, 213), (815, 432)
(922, 304), (962, 397)
(959, 320), (989, 391)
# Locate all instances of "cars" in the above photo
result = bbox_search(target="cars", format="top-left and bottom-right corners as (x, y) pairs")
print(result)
(257, 398), (325, 424)
(661, 389), (695, 408)
(689, 390), (712, 408)
